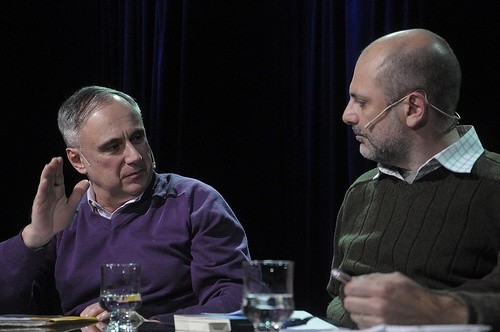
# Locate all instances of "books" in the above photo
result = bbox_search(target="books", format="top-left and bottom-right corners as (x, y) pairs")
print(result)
(172, 309), (255, 332)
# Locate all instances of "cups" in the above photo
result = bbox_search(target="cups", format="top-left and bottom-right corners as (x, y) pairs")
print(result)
(241, 259), (294, 332)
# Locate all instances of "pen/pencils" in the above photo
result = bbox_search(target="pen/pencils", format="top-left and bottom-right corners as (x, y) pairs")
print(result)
(331, 268), (351, 284)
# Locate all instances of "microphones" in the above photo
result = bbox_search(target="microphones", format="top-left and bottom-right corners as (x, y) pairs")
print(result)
(364, 88), (419, 129)
(149, 146), (157, 169)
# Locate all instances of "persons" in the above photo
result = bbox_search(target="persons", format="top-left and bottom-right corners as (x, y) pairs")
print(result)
(325, 28), (500, 332)
(0, 84), (267, 332)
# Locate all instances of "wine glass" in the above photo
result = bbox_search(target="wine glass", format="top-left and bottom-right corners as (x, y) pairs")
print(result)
(99, 263), (142, 332)
